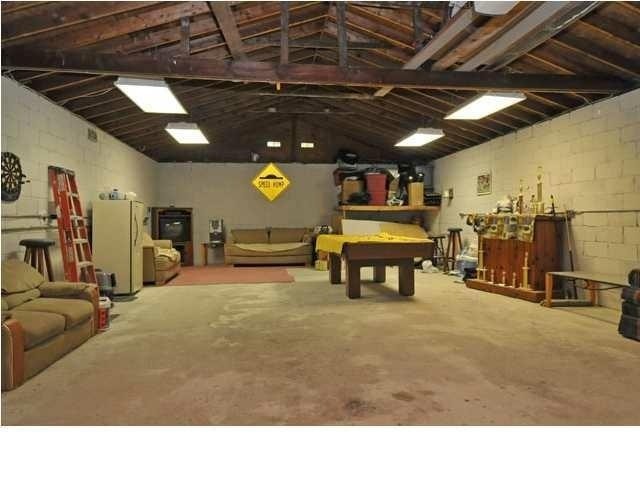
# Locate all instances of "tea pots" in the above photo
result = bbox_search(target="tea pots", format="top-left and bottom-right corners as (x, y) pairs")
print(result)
(110, 190), (136, 200)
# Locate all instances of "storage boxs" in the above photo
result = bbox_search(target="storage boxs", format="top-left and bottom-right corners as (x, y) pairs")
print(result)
(368, 192), (386, 204)
(424, 192), (441, 205)
(413, 164), (434, 185)
(366, 173), (387, 192)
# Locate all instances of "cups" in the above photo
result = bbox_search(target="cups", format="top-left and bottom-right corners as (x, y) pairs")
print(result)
(536, 202), (544, 214)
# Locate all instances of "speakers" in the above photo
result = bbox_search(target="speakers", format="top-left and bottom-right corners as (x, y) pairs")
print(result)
(209, 218), (223, 242)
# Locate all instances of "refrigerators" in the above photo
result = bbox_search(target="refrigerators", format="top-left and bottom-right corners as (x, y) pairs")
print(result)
(89, 198), (144, 294)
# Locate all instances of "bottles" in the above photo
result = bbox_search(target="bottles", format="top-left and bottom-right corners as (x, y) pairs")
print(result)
(550, 195), (555, 213)
(530, 194), (536, 214)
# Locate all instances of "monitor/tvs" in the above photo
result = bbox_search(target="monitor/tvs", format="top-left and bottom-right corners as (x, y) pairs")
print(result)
(159, 214), (190, 243)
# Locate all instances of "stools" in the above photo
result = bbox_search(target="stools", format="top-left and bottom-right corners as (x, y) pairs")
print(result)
(427, 236), (445, 266)
(443, 228), (462, 272)
(19, 239), (55, 281)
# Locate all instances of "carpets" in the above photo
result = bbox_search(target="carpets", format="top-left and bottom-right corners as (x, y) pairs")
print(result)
(164, 266), (295, 285)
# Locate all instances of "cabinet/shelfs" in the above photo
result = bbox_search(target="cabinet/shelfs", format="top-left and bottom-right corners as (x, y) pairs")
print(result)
(458, 213), (567, 303)
(152, 206), (194, 266)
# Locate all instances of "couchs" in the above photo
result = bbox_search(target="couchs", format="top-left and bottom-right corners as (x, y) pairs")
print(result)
(618, 269), (640, 342)
(143, 232), (181, 285)
(224, 228), (312, 267)
(1, 259), (99, 390)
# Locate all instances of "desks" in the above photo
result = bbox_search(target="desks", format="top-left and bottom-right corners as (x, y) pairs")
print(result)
(315, 234), (434, 299)
(200, 242), (224, 265)
(543, 272), (631, 308)
(336, 206), (440, 219)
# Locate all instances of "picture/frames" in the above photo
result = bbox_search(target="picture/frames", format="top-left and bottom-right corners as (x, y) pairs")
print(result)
(477, 175), (492, 193)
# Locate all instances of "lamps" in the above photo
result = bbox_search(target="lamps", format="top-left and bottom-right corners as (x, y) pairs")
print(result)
(443, 92), (527, 119)
(393, 128), (445, 146)
(114, 77), (189, 114)
(164, 121), (210, 144)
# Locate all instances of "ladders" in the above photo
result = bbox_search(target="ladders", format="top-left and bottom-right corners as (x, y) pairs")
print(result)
(48, 165), (101, 297)
